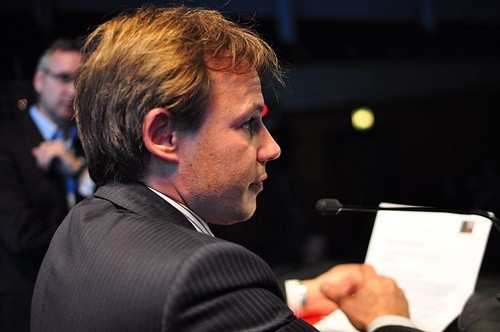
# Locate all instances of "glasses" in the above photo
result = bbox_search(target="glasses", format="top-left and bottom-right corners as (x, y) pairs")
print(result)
(43, 69), (73, 82)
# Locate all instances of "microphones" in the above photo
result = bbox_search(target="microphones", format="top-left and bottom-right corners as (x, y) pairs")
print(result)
(315, 198), (500, 232)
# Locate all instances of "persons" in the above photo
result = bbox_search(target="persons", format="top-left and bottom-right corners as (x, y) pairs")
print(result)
(30, 5), (427, 332)
(0, 37), (97, 332)
